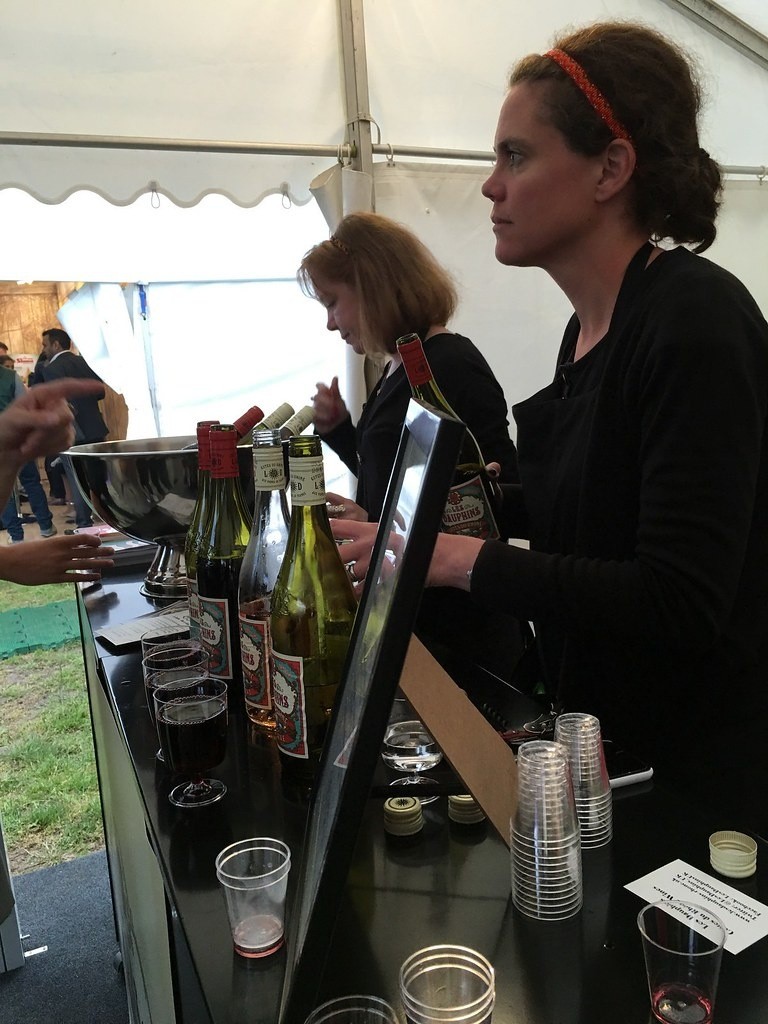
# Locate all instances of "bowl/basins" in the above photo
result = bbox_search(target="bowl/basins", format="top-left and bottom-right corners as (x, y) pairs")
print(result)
(61, 434), (292, 598)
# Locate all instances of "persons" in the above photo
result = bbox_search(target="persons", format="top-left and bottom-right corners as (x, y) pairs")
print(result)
(0, 378), (115, 587)
(0, 328), (110, 546)
(305, 212), (532, 627)
(325, 23), (768, 840)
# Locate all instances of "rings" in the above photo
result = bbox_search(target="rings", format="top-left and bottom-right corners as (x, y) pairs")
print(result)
(346, 564), (359, 582)
(327, 503), (346, 516)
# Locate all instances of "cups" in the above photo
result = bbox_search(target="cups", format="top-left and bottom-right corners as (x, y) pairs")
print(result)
(400, 944), (496, 1024)
(638, 900), (727, 1024)
(510, 740), (583, 921)
(216, 837), (291, 958)
(141, 626), (203, 659)
(554, 713), (613, 849)
(304, 995), (399, 1024)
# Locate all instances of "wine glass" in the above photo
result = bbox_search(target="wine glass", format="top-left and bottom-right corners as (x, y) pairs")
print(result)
(142, 648), (209, 762)
(153, 677), (227, 808)
(381, 699), (444, 806)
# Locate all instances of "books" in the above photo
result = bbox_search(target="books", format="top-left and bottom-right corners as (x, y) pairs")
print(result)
(73, 524), (158, 570)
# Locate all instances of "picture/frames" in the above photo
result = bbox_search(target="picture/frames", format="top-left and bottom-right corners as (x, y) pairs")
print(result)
(277, 399), (518, 1024)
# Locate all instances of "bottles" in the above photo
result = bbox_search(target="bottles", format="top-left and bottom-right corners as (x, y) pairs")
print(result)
(238, 431), (291, 725)
(196, 424), (254, 704)
(186, 420), (221, 627)
(271, 436), (359, 771)
(397, 333), (509, 545)
(236, 402), (295, 446)
(181, 406), (264, 450)
(280, 406), (317, 440)
